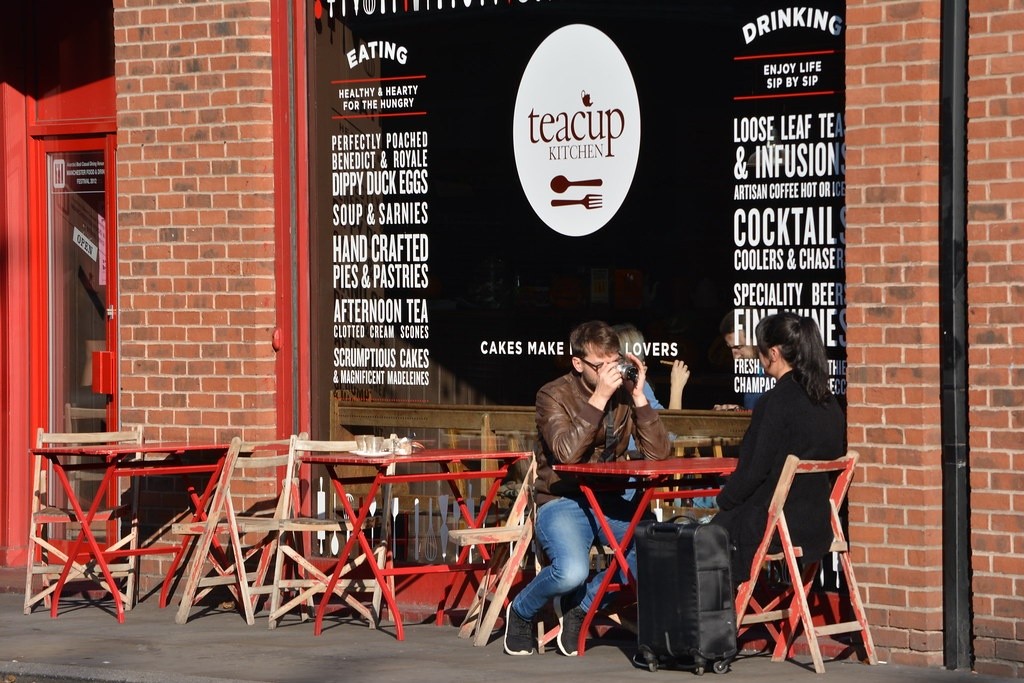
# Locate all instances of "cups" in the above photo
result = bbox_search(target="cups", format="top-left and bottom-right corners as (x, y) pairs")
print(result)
(394, 439), (412, 456)
(355, 434), (385, 454)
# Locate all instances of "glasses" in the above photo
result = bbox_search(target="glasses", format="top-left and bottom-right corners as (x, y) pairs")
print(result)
(580, 351), (624, 373)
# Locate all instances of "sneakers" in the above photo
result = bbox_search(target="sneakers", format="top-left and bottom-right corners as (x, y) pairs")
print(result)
(504, 601), (533, 655)
(553, 595), (587, 657)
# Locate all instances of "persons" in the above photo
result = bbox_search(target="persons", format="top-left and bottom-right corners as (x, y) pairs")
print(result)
(698, 314), (847, 590)
(674, 307), (778, 510)
(502, 319), (672, 657)
(612, 324), (691, 507)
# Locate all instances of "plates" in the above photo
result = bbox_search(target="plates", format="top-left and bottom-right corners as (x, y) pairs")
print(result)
(349, 450), (391, 458)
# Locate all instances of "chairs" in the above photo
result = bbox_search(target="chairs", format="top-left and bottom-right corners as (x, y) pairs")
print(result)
(22, 400), (879, 675)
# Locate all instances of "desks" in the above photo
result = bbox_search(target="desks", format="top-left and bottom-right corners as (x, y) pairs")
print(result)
(552, 457), (739, 656)
(25, 441), (232, 623)
(298, 451), (533, 640)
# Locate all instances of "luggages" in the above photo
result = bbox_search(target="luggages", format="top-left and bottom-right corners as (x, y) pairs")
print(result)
(633, 515), (737, 675)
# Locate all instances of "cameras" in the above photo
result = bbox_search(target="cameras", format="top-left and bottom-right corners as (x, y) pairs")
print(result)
(617, 365), (638, 381)
